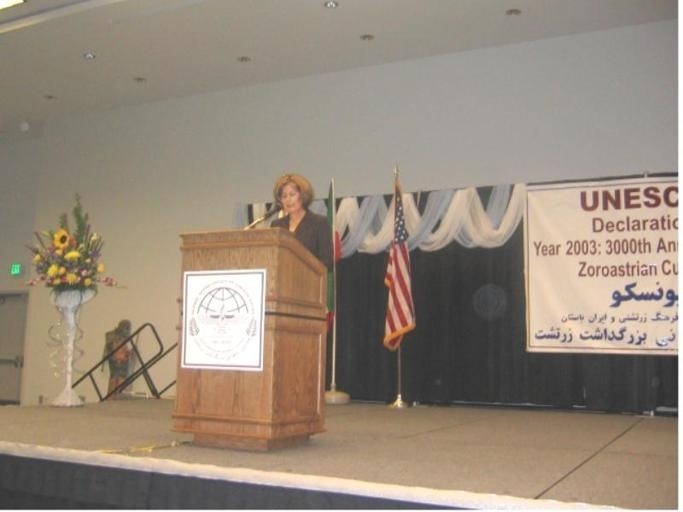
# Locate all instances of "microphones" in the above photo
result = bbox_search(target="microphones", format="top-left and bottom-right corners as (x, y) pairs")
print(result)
(238, 202), (283, 228)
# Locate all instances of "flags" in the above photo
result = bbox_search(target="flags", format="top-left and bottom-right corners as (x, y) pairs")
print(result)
(320, 181), (344, 334)
(380, 179), (416, 352)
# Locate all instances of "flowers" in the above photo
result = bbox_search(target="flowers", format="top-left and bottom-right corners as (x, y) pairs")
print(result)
(21, 194), (118, 288)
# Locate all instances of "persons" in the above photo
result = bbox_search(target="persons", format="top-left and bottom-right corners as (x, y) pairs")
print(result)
(269, 173), (335, 272)
(104, 319), (132, 399)
